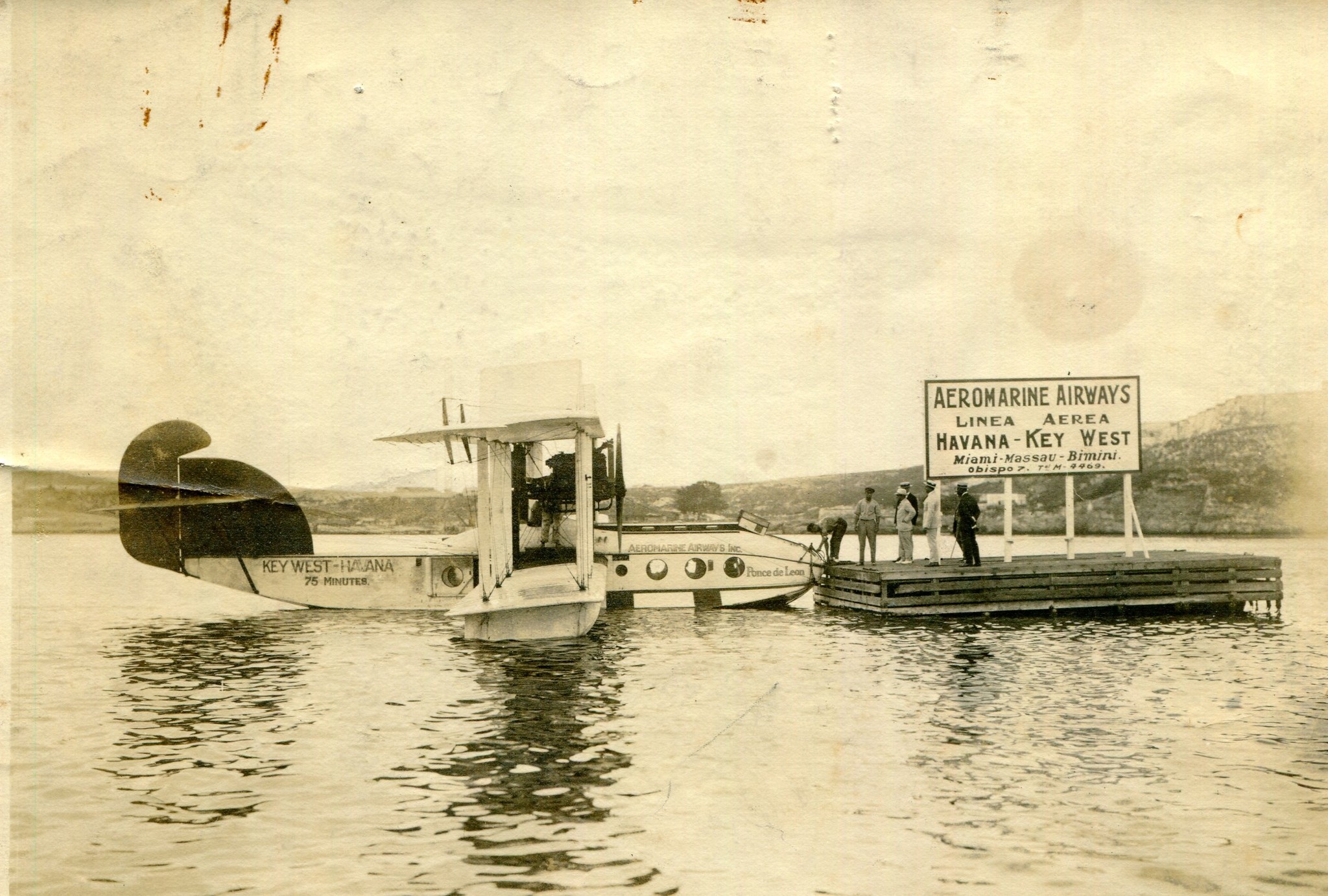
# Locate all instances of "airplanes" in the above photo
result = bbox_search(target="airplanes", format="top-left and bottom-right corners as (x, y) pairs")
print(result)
(89, 356), (829, 640)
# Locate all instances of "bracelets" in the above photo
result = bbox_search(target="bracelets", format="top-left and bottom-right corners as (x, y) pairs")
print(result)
(876, 522), (879, 525)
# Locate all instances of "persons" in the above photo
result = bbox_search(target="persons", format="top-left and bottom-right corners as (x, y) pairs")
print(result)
(892, 481), (918, 565)
(922, 479), (941, 567)
(853, 486), (882, 566)
(807, 515), (848, 563)
(952, 482), (981, 568)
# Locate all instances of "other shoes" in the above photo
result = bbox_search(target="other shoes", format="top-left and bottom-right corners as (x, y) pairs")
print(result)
(958, 559), (967, 563)
(924, 562), (939, 567)
(893, 557), (901, 562)
(857, 562), (864, 565)
(872, 562), (877, 565)
(959, 563), (973, 567)
(896, 558), (905, 564)
(901, 560), (912, 564)
(974, 562), (982, 567)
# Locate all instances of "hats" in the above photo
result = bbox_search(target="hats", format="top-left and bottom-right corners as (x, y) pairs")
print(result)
(897, 481), (913, 488)
(865, 487), (875, 492)
(923, 479), (937, 489)
(894, 488), (908, 495)
(954, 480), (969, 489)
